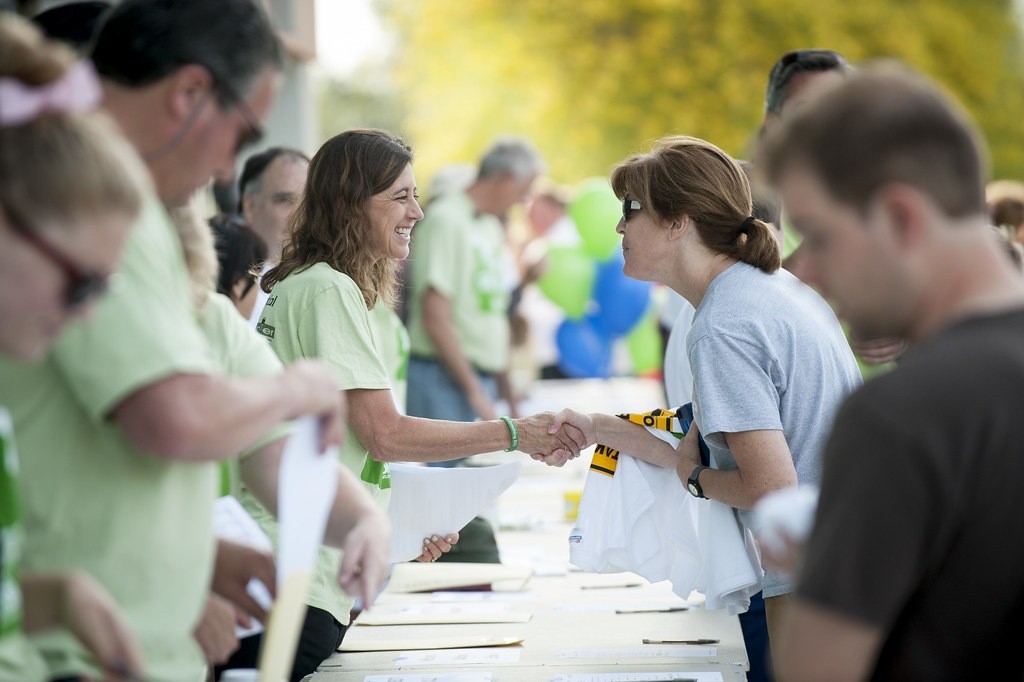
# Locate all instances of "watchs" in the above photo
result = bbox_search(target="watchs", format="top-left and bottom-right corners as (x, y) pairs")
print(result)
(687, 466), (712, 501)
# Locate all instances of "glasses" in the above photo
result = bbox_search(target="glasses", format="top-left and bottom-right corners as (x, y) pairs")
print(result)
(0, 204), (109, 312)
(623, 198), (647, 221)
(248, 271), (265, 284)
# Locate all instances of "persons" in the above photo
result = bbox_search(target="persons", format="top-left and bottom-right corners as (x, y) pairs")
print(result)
(727, 46), (1024, 384)
(748, 70), (1024, 682)
(529, 136), (866, 682)
(0, 2), (587, 682)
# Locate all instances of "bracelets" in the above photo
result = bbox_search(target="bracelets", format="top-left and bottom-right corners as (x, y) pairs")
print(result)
(499, 416), (518, 452)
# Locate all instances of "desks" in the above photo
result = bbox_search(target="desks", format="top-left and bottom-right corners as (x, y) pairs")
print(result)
(298, 379), (751, 682)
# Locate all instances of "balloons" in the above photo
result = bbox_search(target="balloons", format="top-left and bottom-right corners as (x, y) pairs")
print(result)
(536, 174), (651, 380)
